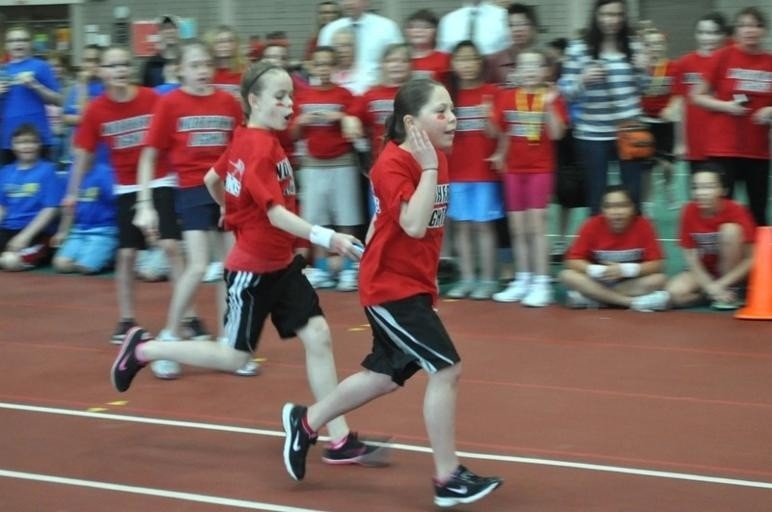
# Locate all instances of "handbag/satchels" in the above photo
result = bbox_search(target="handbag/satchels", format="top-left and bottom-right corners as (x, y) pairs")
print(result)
(616, 116), (661, 165)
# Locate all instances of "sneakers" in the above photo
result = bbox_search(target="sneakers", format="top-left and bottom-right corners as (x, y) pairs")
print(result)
(281, 401), (318, 483)
(322, 429), (388, 465)
(468, 278), (500, 302)
(564, 288), (601, 312)
(630, 289), (671, 314)
(108, 314), (210, 394)
(446, 279), (475, 300)
(712, 288), (745, 309)
(521, 279), (557, 309)
(135, 249), (359, 296)
(233, 361), (260, 376)
(432, 465), (503, 508)
(491, 275), (528, 304)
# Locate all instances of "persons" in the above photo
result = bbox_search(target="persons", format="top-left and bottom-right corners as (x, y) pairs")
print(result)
(281, 80), (506, 507)
(110, 61), (394, 464)
(131, 36), (262, 380)
(60, 43), (210, 342)
(2, 0), (771, 313)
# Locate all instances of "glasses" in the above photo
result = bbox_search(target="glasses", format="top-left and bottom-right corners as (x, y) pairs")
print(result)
(95, 56), (134, 71)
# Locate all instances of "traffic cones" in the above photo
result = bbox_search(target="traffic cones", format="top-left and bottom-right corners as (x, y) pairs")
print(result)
(733, 225), (772, 320)
